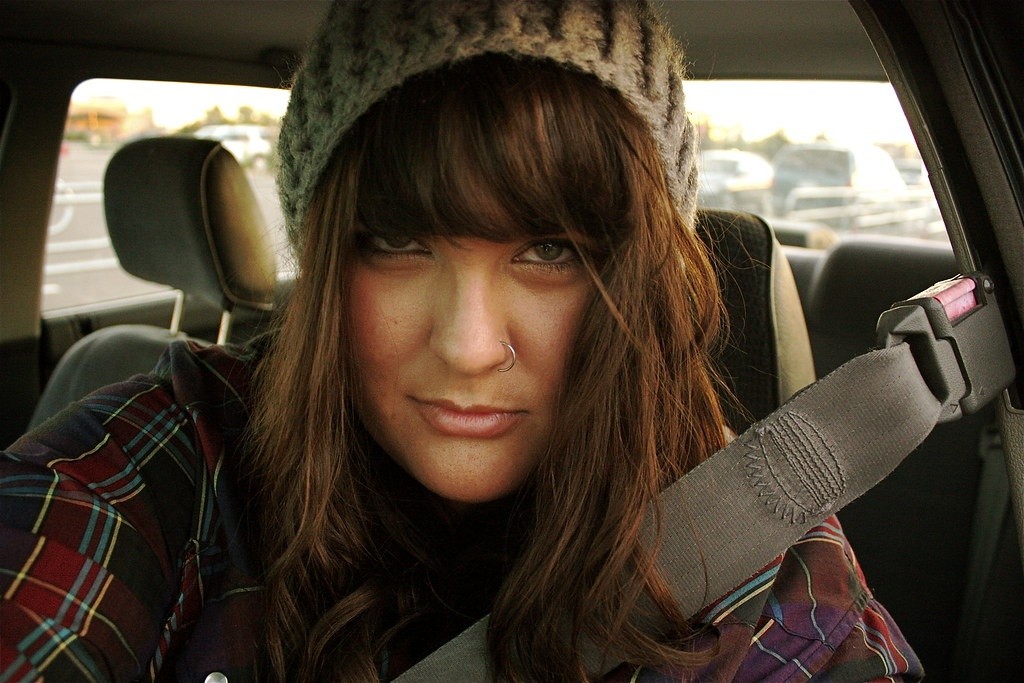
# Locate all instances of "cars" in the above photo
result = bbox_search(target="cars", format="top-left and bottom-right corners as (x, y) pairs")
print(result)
(194, 124), (275, 171)
(688, 148), (773, 196)
(891, 160), (928, 187)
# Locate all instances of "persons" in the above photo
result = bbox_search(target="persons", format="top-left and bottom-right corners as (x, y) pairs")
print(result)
(0, 0), (930, 683)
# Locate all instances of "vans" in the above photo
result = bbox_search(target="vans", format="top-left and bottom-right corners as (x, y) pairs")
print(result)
(768, 141), (907, 212)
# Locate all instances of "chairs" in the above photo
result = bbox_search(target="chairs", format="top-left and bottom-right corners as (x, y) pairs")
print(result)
(28, 136), (279, 436)
(693, 206), (815, 437)
(809, 233), (1008, 674)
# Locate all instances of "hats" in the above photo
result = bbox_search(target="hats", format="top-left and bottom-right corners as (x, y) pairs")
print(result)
(275, 0), (697, 254)
